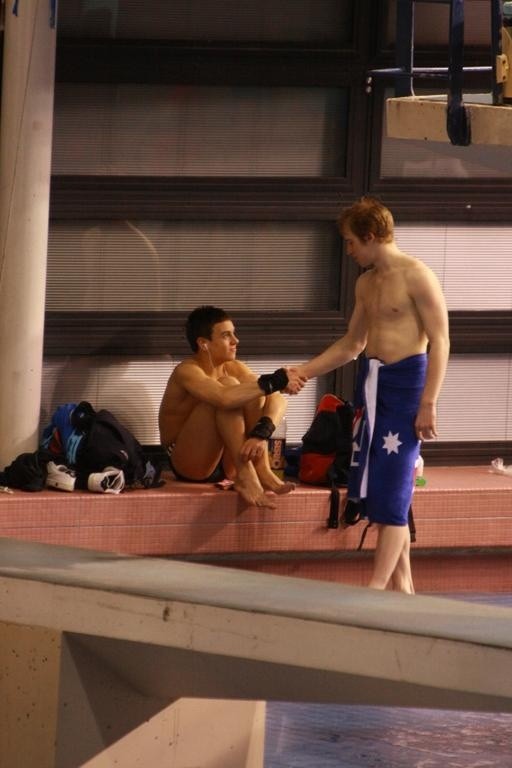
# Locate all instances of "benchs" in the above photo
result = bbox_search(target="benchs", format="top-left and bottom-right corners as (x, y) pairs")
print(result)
(3, 466), (511, 558)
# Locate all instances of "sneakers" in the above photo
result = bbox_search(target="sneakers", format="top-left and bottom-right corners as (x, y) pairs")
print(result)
(45, 459), (77, 492)
(87, 466), (126, 495)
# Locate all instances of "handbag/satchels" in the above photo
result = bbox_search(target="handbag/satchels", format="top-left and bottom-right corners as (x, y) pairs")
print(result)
(297, 393), (365, 485)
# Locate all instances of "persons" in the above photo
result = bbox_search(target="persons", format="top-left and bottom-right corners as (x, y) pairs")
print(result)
(277, 196), (453, 596)
(157, 303), (309, 508)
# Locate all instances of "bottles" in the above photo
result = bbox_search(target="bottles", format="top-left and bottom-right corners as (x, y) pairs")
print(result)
(267, 414), (287, 483)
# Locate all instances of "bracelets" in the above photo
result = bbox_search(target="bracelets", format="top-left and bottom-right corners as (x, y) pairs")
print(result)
(249, 416), (276, 440)
(258, 369), (288, 396)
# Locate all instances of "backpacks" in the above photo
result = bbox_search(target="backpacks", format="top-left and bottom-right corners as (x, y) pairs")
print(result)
(71, 400), (166, 488)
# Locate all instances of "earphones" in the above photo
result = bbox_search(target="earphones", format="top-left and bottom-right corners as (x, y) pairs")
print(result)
(202, 343), (208, 349)
